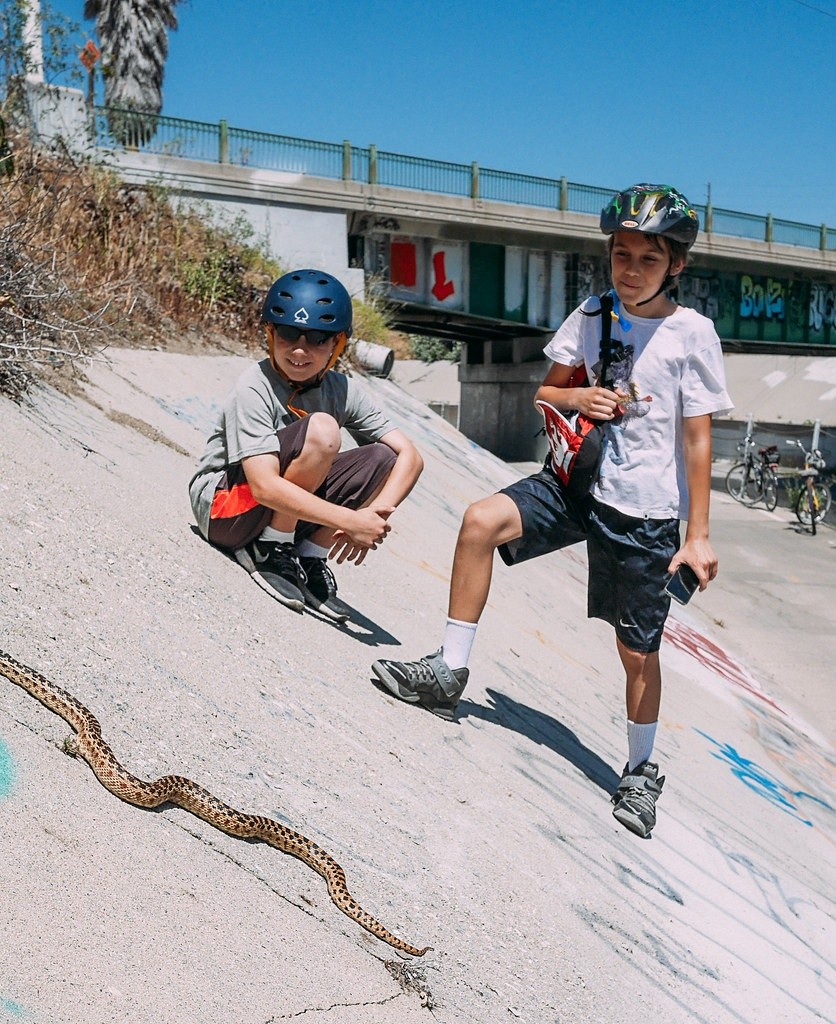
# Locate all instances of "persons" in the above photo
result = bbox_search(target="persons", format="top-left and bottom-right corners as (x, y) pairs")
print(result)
(189, 269), (424, 625)
(373, 183), (736, 836)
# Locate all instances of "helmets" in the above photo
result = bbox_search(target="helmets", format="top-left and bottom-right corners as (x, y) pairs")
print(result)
(600, 183), (699, 251)
(260, 269), (354, 340)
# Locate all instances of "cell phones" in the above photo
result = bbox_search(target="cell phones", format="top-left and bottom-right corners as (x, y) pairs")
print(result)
(665, 563), (699, 606)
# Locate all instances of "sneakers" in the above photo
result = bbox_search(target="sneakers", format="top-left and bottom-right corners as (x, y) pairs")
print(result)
(234, 537), (308, 611)
(370, 646), (469, 721)
(294, 556), (350, 625)
(608, 760), (666, 838)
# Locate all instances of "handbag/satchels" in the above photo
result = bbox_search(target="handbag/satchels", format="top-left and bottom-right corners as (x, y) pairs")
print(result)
(536, 294), (614, 501)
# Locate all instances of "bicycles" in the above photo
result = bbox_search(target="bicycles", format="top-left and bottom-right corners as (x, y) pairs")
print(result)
(785, 439), (831, 535)
(725, 432), (782, 513)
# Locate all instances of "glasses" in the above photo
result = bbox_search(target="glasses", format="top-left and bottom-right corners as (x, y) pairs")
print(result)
(270, 322), (340, 346)
